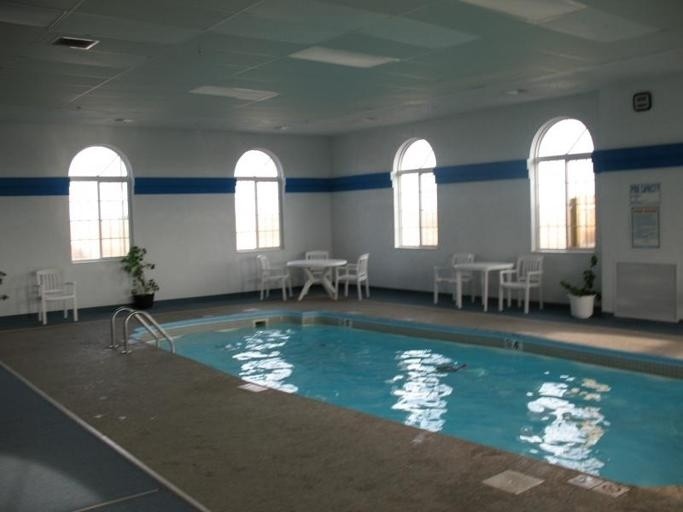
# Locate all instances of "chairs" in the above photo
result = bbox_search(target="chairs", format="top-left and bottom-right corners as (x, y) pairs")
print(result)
(32, 267), (86, 326)
(497, 250), (547, 316)
(256, 252), (298, 304)
(429, 251), (480, 312)
(304, 248), (338, 299)
(335, 250), (376, 304)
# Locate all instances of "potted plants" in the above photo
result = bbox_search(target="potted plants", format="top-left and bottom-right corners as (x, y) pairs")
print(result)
(559, 251), (604, 324)
(118, 241), (162, 309)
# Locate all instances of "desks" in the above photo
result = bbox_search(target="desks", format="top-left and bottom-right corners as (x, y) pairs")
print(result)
(285, 257), (350, 304)
(449, 261), (518, 316)
